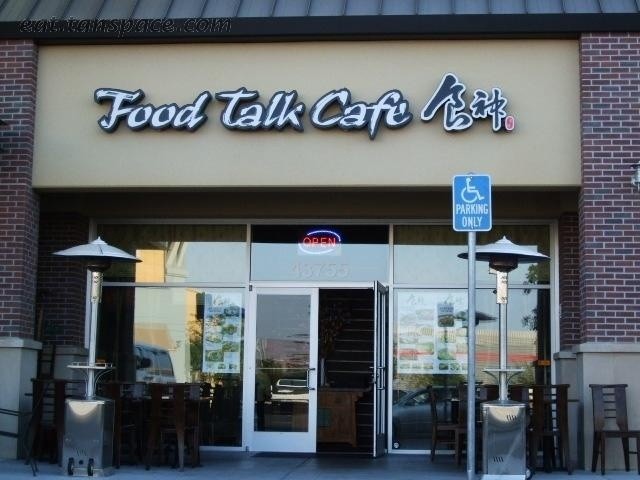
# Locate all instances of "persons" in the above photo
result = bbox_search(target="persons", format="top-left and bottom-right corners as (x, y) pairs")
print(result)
(255, 364), (272, 430)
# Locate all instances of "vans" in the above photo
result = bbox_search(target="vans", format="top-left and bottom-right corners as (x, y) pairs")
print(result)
(136, 345), (176, 384)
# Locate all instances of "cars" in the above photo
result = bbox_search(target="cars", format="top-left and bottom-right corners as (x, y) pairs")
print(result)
(392, 386), (456, 439)
(271, 379), (308, 403)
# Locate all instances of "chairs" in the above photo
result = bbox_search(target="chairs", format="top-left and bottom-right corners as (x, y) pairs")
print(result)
(426, 379), (640, 476)
(0, 377), (209, 477)
